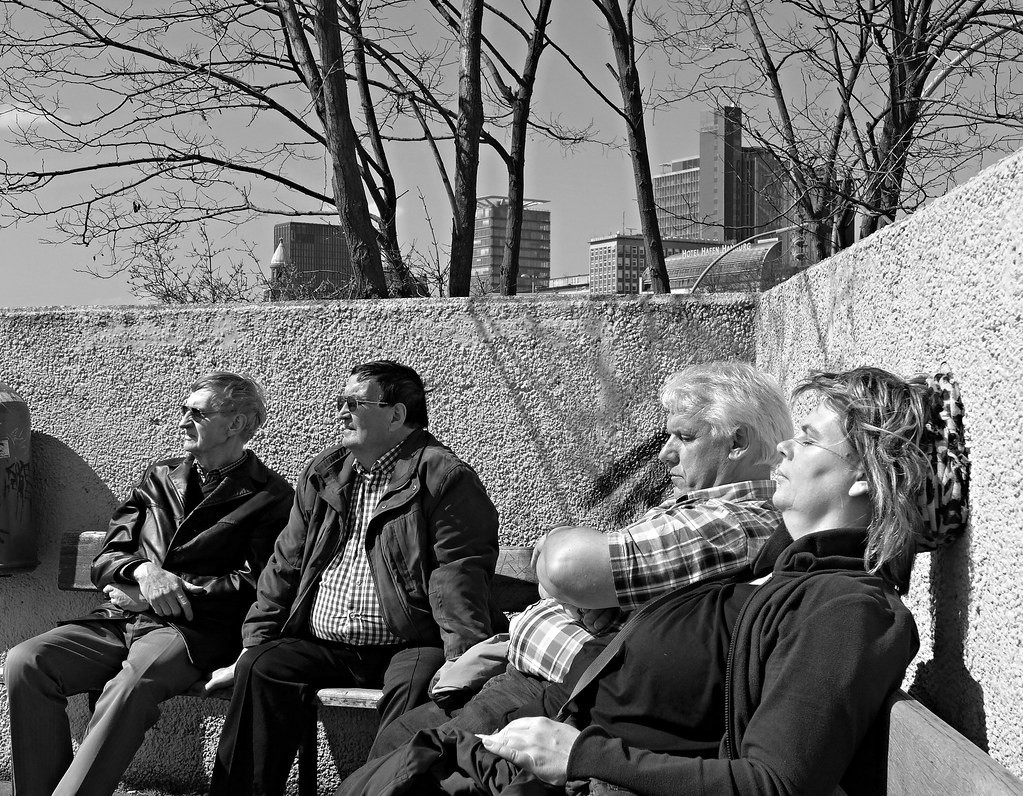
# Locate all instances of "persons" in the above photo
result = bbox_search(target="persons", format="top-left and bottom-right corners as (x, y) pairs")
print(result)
(202, 362), (500, 796)
(6, 372), (295, 796)
(366, 360), (969, 796)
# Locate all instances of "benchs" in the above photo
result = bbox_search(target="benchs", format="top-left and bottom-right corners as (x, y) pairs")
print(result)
(51, 530), (1023, 796)
